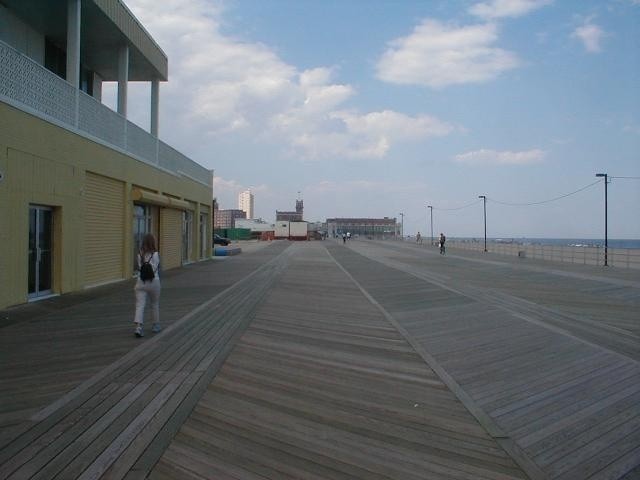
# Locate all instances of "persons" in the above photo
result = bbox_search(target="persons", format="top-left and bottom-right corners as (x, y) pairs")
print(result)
(130, 233), (162, 339)
(341, 231), (347, 244)
(416, 231), (420, 245)
(438, 233), (446, 255)
(346, 231), (351, 240)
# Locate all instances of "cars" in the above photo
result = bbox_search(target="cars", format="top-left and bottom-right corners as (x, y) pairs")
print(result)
(213, 234), (231, 246)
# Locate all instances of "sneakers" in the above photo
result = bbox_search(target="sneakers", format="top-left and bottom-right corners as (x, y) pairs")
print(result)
(152, 324), (161, 332)
(134, 327), (144, 337)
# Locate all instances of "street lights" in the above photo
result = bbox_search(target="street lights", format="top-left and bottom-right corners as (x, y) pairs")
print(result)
(428, 206), (434, 245)
(479, 196), (487, 252)
(400, 213), (403, 239)
(596, 173), (608, 267)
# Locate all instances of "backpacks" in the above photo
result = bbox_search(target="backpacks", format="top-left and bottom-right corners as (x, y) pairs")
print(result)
(140, 253), (155, 281)
